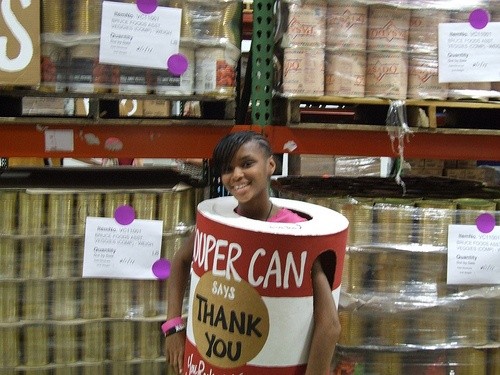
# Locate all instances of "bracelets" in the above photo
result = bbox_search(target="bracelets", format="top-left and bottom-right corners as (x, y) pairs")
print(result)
(161, 316), (186, 336)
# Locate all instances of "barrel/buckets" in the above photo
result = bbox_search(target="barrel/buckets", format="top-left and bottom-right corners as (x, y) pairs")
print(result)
(1, 188), (201, 375)
(274, 0), (499, 104)
(22, 0), (241, 100)
(286, 191), (500, 375)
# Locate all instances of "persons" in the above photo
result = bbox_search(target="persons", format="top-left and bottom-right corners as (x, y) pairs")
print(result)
(163, 130), (341, 374)
